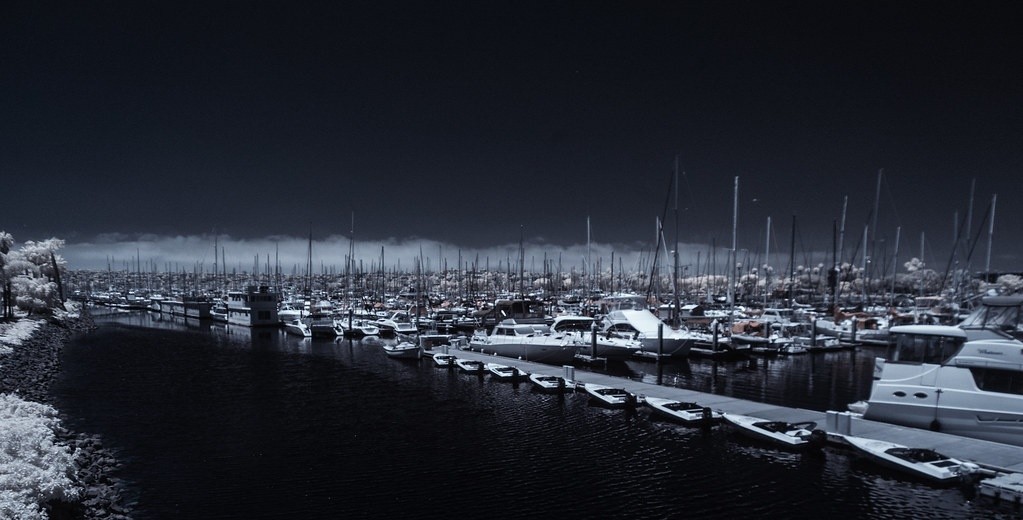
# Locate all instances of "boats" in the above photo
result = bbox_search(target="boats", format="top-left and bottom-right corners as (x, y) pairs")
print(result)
(842, 434), (981, 487)
(455, 358), (486, 372)
(488, 362), (531, 381)
(584, 382), (646, 408)
(528, 373), (577, 393)
(842, 325), (1023, 448)
(721, 411), (829, 450)
(978, 472), (1023, 505)
(432, 352), (458, 367)
(645, 396), (726, 425)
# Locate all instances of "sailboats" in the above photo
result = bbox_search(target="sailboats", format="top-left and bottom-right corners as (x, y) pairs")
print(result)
(71, 153), (1023, 366)
(382, 259), (425, 360)
